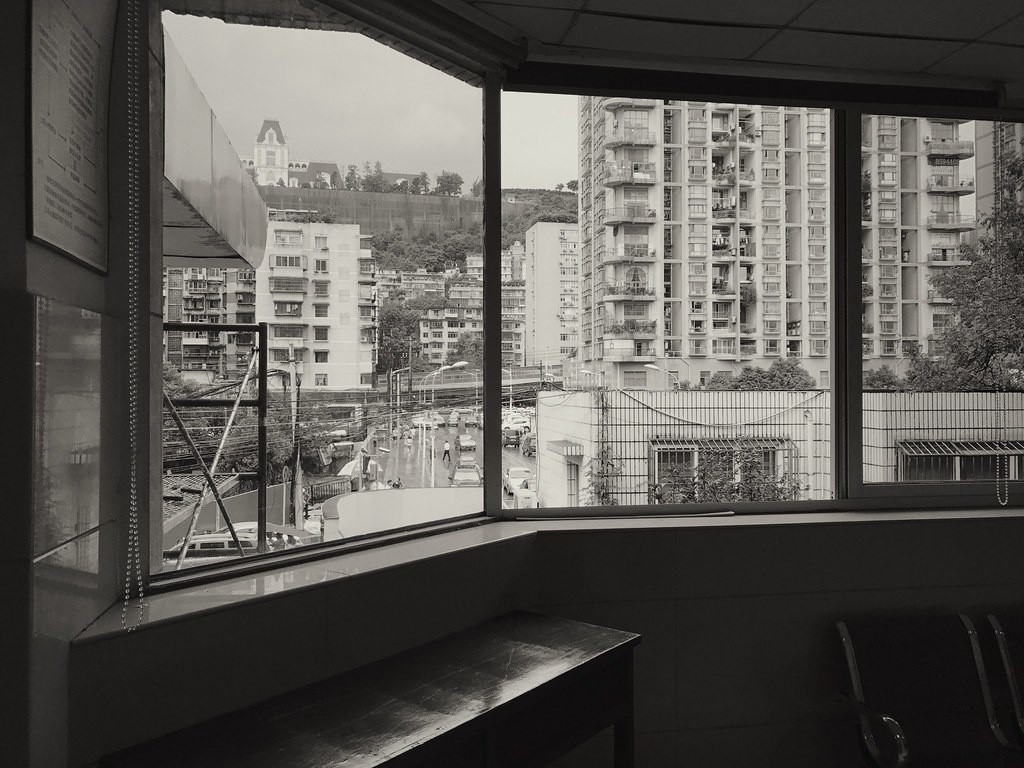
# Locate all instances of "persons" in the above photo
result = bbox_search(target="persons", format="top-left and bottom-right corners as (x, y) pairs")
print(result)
(386, 480), (393, 489)
(286, 535), (296, 546)
(393, 477), (402, 488)
(268, 533), (285, 551)
(393, 421), (416, 454)
(442, 441), (451, 461)
(430, 446), (436, 466)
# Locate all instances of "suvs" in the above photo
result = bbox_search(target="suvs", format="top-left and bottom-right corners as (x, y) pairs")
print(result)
(521, 436), (536, 456)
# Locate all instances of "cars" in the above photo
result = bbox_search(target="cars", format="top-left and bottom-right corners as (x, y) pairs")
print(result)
(502, 418), (531, 433)
(448, 418), (459, 427)
(502, 405), (536, 423)
(503, 467), (533, 495)
(454, 407), (474, 414)
(411, 407), (452, 430)
(465, 416), (478, 427)
(449, 461), (483, 487)
(513, 475), (539, 509)
(454, 435), (477, 451)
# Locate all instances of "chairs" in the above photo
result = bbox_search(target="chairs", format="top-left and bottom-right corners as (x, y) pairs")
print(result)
(827, 603), (1024, 768)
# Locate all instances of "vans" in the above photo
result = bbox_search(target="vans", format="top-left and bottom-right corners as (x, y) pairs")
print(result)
(501, 429), (520, 448)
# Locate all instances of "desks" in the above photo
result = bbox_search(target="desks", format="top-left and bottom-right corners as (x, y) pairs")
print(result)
(100, 608), (643, 768)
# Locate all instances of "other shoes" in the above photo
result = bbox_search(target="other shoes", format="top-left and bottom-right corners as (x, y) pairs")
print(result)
(442, 459), (445, 460)
(448, 459), (451, 460)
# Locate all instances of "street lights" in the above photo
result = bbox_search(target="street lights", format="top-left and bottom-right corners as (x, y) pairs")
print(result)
(417, 360), (483, 487)
(389, 367), (412, 455)
